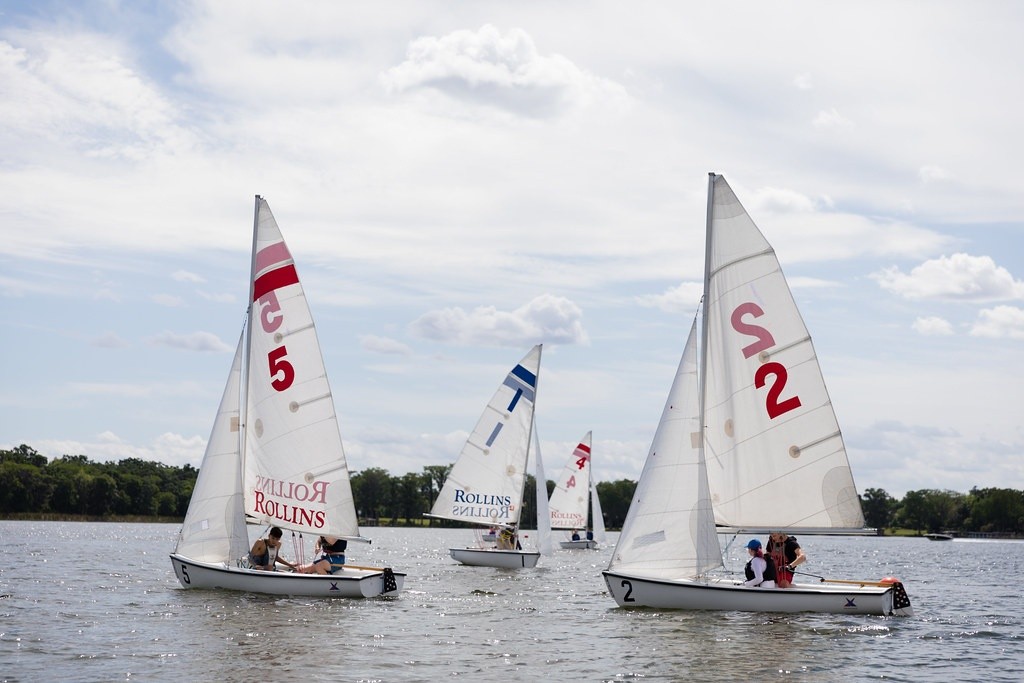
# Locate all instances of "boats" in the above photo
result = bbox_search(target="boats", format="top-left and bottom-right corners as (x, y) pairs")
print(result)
(922, 534), (953, 540)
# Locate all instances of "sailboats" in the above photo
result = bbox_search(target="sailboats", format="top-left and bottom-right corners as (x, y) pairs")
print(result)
(544, 429), (609, 550)
(168, 195), (407, 599)
(420, 343), (544, 571)
(596, 169), (918, 614)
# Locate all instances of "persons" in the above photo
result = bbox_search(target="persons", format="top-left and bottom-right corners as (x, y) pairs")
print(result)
(586, 527), (593, 540)
(298, 537), (347, 575)
(248, 527), (302, 571)
(743, 539), (775, 588)
(490, 523), (522, 551)
(765, 533), (807, 588)
(572, 530), (580, 541)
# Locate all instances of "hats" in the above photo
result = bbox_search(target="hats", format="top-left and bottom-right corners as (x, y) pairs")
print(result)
(744, 539), (762, 550)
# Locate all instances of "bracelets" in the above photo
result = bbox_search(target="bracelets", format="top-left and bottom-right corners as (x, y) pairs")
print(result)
(789, 564), (792, 568)
